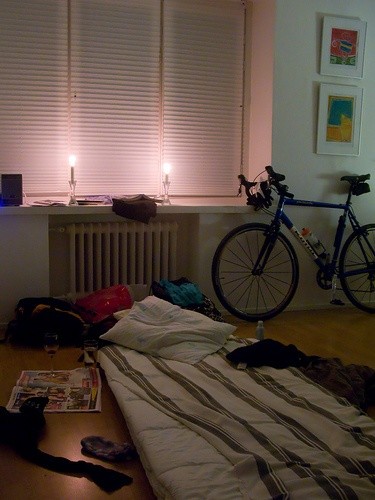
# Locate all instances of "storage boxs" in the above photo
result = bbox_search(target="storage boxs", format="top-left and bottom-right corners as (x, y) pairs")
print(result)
(1, 174), (23, 207)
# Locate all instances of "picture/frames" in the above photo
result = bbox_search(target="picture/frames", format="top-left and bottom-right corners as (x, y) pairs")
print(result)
(315, 82), (364, 157)
(319, 15), (367, 79)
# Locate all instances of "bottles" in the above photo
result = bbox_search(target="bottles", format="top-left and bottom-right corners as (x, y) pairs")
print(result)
(255, 321), (264, 341)
(301, 228), (328, 260)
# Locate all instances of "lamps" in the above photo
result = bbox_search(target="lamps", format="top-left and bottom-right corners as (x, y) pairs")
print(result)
(161, 162), (172, 205)
(67, 155), (78, 206)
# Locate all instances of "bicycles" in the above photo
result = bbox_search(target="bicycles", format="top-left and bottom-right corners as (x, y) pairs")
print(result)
(211, 165), (375, 322)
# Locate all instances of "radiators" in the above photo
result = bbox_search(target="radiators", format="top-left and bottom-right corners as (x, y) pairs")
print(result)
(49, 222), (179, 304)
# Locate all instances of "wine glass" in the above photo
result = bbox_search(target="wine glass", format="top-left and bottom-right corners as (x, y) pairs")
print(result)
(44, 334), (60, 377)
(82, 340), (98, 385)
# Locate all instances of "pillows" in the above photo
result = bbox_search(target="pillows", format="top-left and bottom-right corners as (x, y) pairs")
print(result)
(100, 296), (239, 365)
